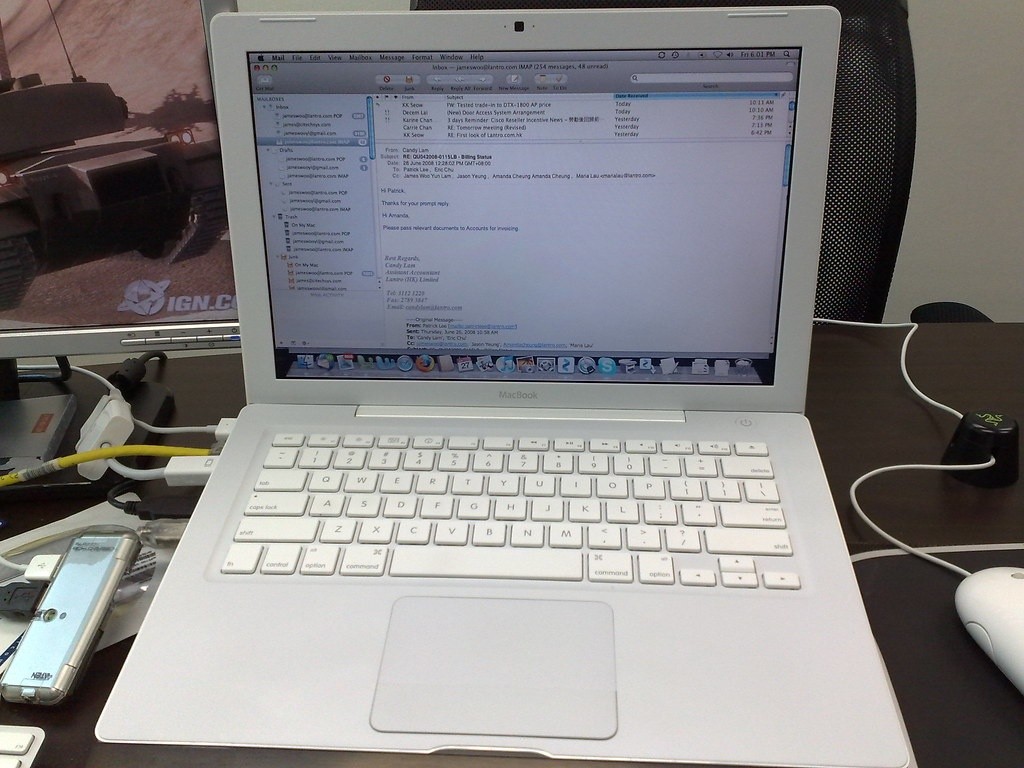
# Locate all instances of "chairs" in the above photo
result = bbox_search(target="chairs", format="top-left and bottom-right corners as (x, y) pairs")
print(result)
(408, 0), (920, 326)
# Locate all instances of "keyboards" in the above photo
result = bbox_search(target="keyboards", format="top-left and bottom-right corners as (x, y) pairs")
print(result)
(0, 724), (45, 768)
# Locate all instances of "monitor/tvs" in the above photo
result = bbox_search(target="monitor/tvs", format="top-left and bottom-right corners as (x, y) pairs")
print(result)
(0, 0), (253, 502)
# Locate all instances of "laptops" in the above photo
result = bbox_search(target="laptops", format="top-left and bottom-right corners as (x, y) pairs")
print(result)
(93, 6), (918, 768)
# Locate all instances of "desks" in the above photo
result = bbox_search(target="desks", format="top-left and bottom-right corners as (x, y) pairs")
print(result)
(0, 361), (1024, 768)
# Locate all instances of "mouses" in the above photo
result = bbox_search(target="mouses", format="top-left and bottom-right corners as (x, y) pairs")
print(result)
(955, 567), (1024, 696)
